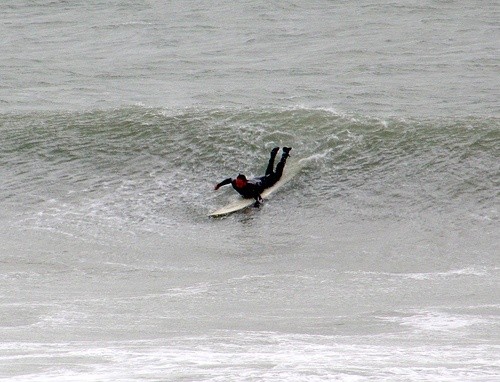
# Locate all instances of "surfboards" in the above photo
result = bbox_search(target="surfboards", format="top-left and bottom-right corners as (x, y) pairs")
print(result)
(210, 162), (302, 216)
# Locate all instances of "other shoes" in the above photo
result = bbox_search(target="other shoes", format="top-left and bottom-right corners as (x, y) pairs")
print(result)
(283, 147), (291, 152)
(272, 147), (279, 152)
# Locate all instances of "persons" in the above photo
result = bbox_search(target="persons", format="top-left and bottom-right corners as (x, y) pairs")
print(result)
(215, 146), (292, 207)
